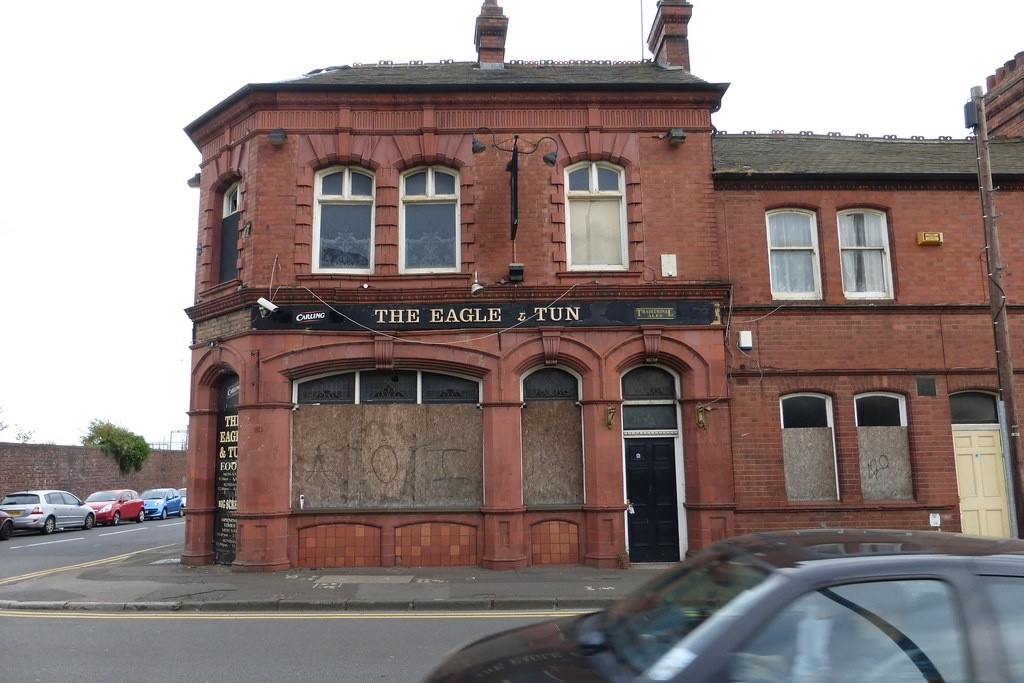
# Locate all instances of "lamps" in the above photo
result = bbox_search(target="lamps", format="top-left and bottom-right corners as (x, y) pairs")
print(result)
(268, 128), (287, 146)
(187, 173), (200, 187)
(470, 269), (484, 298)
(470, 126), (559, 166)
(663, 128), (686, 144)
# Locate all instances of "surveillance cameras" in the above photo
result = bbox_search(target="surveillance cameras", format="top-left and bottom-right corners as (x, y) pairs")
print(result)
(257, 298), (279, 312)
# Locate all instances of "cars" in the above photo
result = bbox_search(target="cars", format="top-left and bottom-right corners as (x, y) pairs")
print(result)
(420, 522), (1024, 683)
(178, 487), (188, 506)
(85, 489), (145, 526)
(0, 489), (97, 535)
(139, 487), (185, 520)
(0, 510), (14, 540)
